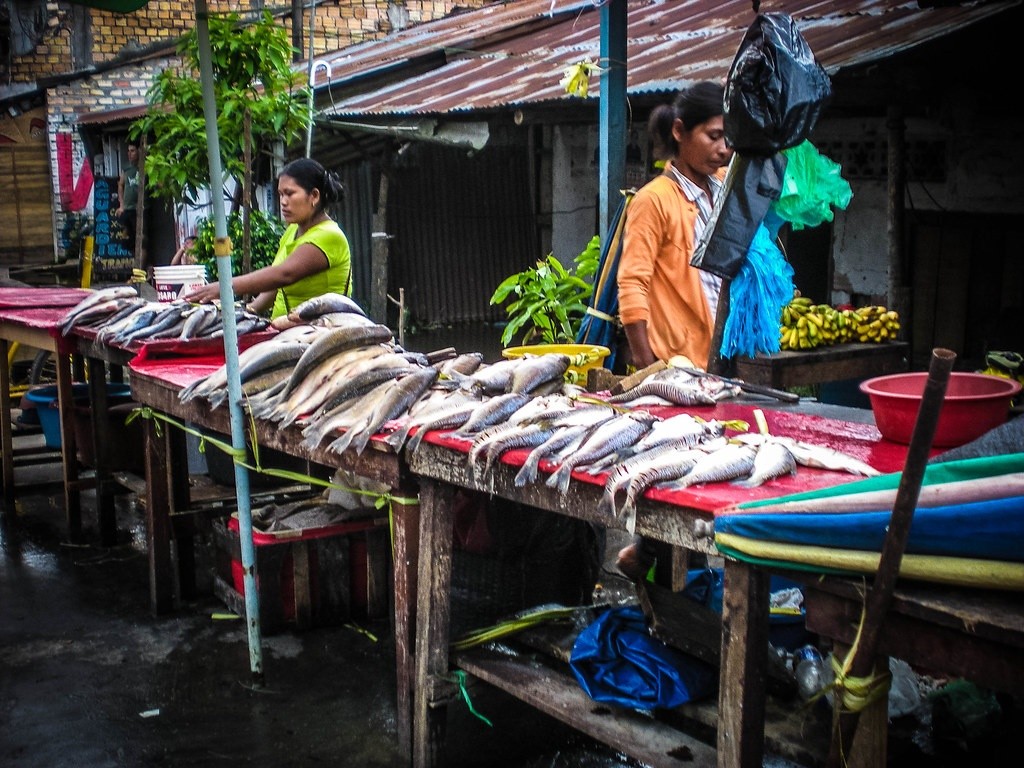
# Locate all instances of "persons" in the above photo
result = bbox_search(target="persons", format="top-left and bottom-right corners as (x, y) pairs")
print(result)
(614, 80), (733, 583)
(182, 158), (355, 315)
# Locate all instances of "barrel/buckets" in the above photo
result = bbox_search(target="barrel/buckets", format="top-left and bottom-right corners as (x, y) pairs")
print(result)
(153, 265), (209, 302)
(54, 393), (140, 466)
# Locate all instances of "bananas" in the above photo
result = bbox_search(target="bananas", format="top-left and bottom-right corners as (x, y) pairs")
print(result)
(776, 294), (900, 349)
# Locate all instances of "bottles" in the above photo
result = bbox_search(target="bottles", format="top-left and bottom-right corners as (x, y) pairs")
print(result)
(792, 645), (826, 700)
(591, 583), (607, 606)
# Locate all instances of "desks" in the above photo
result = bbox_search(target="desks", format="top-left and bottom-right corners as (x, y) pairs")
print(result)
(128, 353), (1024, 768)
(0, 318), (280, 547)
(735, 340), (911, 391)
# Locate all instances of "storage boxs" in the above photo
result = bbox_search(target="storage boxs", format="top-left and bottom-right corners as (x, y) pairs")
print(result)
(452, 543), (560, 615)
(212, 517), (391, 634)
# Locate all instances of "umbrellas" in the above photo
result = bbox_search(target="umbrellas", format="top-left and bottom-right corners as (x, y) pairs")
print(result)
(694, 418), (1023, 593)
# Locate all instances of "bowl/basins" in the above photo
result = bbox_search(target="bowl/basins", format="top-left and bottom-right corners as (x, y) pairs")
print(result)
(501, 344), (611, 388)
(858, 372), (1023, 447)
(24, 383), (131, 450)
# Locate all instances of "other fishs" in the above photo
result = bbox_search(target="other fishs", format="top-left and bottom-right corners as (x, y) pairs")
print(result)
(58, 284), (885, 538)
